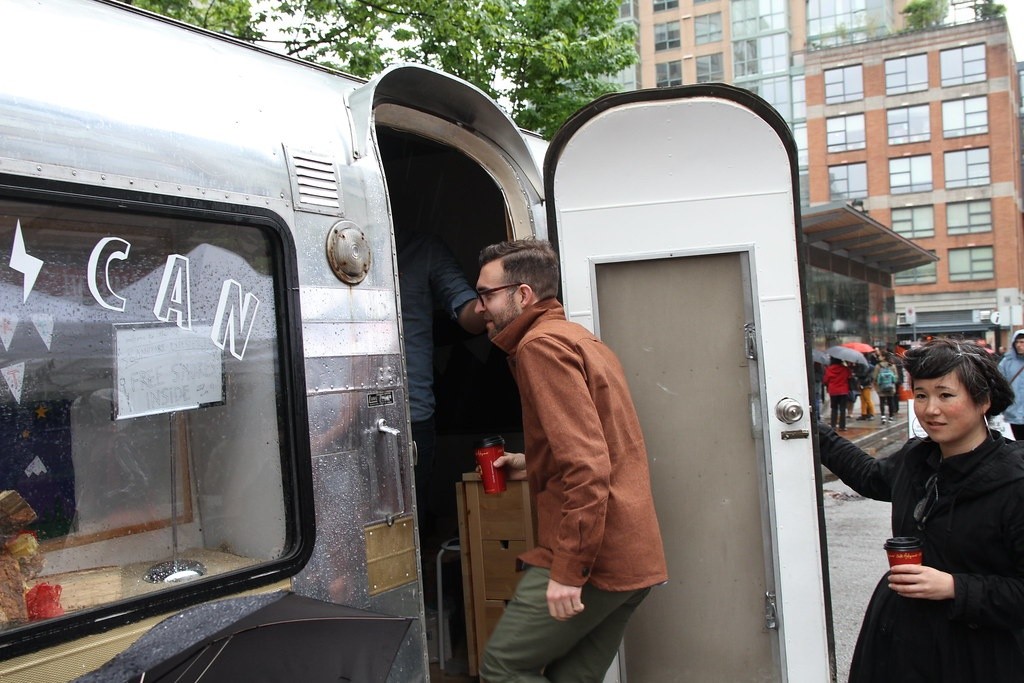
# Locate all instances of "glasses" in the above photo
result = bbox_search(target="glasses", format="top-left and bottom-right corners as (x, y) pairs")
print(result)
(476, 284), (523, 306)
(913, 473), (941, 526)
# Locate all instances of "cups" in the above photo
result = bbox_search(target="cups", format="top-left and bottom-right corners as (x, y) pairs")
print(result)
(472, 434), (507, 493)
(883, 537), (923, 586)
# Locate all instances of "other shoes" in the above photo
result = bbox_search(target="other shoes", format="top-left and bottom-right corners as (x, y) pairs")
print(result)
(881, 416), (886, 424)
(869, 415), (874, 421)
(857, 416), (866, 420)
(888, 418), (896, 423)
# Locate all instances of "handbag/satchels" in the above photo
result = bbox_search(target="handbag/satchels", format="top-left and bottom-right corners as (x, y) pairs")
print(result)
(847, 378), (861, 402)
(107, 434), (158, 529)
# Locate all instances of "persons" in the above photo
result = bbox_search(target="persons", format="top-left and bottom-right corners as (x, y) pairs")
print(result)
(816, 339), (1024, 683)
(998, 329), (1024, 440)
(812, 346), (904, 432)
(393, 227), (488, 506)
(475, 239), (667, 683)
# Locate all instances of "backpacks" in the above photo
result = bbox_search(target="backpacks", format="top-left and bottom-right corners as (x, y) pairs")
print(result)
(877, 362), (896, 392)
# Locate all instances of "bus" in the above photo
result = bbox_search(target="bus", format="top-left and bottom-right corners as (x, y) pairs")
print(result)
(0, 1), (839, 683)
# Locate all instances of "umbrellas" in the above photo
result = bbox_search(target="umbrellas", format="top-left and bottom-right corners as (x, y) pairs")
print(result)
(812, 348), (830, 366)
(841, 340), (875, 354)
(826, 345), (869, 369)
